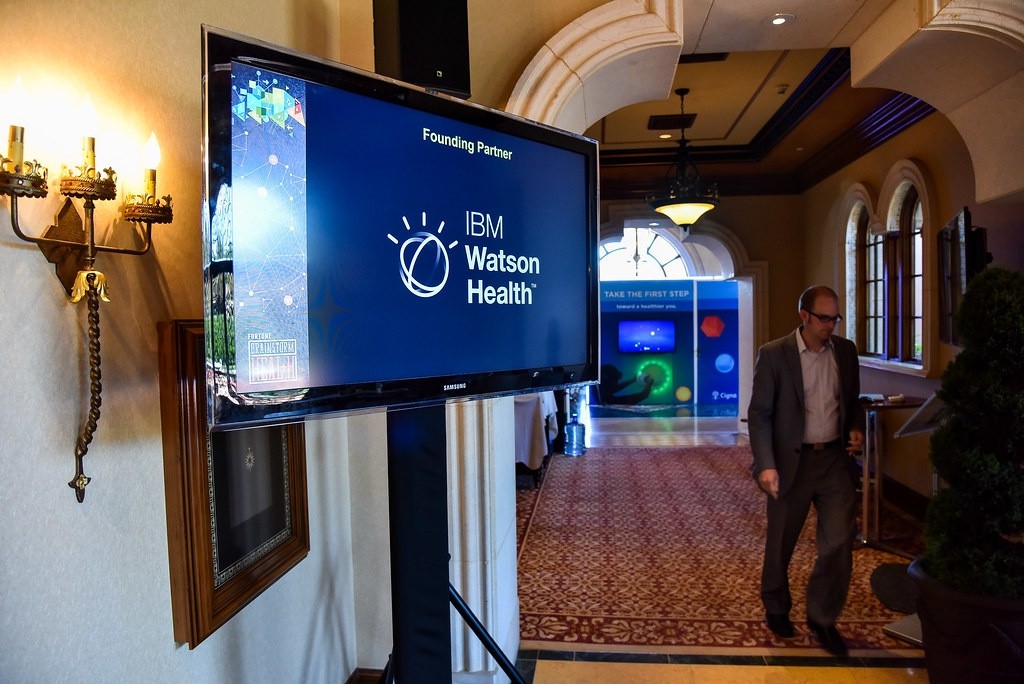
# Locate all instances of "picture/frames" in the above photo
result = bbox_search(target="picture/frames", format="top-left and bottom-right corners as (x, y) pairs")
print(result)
(157, 317), (311, 650)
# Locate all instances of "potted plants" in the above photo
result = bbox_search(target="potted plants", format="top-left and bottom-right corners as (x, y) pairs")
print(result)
(905, 268), (1024, 684)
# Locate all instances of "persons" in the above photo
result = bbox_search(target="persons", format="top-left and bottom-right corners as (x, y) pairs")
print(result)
(747, 285), (866, 657)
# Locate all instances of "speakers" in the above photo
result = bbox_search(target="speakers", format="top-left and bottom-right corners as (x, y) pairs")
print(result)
(372, 0), (470, 101)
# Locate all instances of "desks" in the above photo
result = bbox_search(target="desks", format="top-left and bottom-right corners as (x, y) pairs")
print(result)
(516, 387), (560, 487)
(850, 392), (929, 561)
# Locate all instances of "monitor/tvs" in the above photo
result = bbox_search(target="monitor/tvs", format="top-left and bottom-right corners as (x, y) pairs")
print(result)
(617, 319), (677, 354)
(198, 24), (599, 431)
(936, 206), (989, 348)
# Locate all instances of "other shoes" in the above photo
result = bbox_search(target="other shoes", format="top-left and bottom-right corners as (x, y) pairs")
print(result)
(807, 616), (848, 656)
(766, 613), (794, 637)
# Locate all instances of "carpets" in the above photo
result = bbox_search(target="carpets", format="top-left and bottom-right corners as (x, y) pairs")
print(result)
(514, 445), (942, 649)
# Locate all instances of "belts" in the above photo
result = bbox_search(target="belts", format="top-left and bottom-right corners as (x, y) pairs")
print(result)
(801, 437), (842, 450)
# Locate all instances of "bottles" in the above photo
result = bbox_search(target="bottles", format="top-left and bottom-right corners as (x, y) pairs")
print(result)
(564, 414), (585, 457)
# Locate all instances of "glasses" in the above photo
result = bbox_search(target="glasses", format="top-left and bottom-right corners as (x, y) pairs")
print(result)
(808, 311), (843, 323)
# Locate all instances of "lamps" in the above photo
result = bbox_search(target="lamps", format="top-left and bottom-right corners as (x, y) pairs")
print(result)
(0, 93), (175, 502)
(640, 88), (722, 232)
